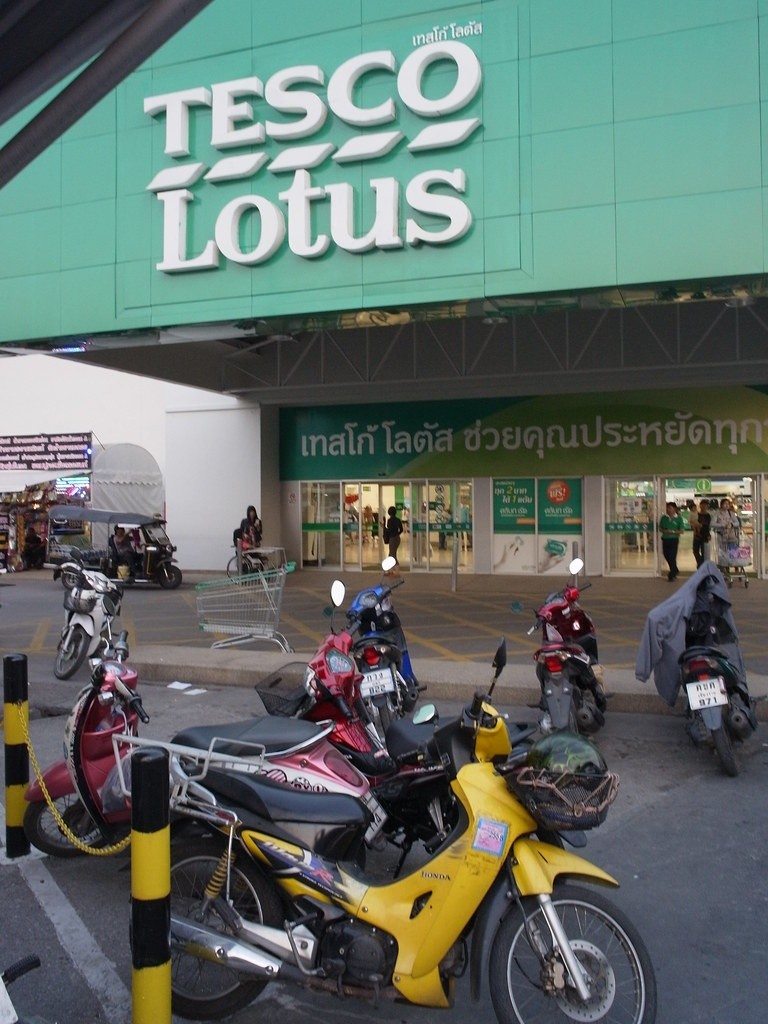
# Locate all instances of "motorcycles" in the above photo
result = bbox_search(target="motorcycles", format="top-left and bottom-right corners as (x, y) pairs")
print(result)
(251, 579), (591, 904)
(47, 503), (184, 590)
(44, 546), (126, 681)
(527, 556), (616, 742)
(21, 625), (390, 919)
(678, 611), (755, 778)
(324, 554), (430, 736)
(113, 628), (662, 1024)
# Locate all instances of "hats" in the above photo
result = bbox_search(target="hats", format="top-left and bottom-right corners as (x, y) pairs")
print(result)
(700, 500), (709, 505)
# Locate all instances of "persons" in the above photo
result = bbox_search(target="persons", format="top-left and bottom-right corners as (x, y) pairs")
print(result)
(709, 499), (742, 574)
(240, 505), (263, 572)
(689, 500), (712, 570)
(21, 528), (43, 568)
(114, 525), (137, 579)
(659, 502), (684, 582)
(380, 506), (403, 567)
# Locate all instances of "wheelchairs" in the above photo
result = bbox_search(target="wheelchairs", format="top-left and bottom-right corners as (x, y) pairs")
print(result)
(225, 545), (280, 586)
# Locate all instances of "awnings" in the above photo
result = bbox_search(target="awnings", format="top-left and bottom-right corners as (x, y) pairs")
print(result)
(0, 471), (92, 491)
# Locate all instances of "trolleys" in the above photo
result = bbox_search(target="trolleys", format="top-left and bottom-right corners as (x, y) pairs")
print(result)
(192, 560), (296, 656)
(713, 524), (753, 589)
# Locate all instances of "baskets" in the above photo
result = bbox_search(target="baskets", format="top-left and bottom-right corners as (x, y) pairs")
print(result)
(503, 766), (618, 832)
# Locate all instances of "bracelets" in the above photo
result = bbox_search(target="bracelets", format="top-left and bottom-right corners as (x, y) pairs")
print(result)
(672, 530), (675, 534)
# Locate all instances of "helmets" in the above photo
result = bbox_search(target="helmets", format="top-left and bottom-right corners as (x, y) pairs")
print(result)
(524, 729), (609, 799)
